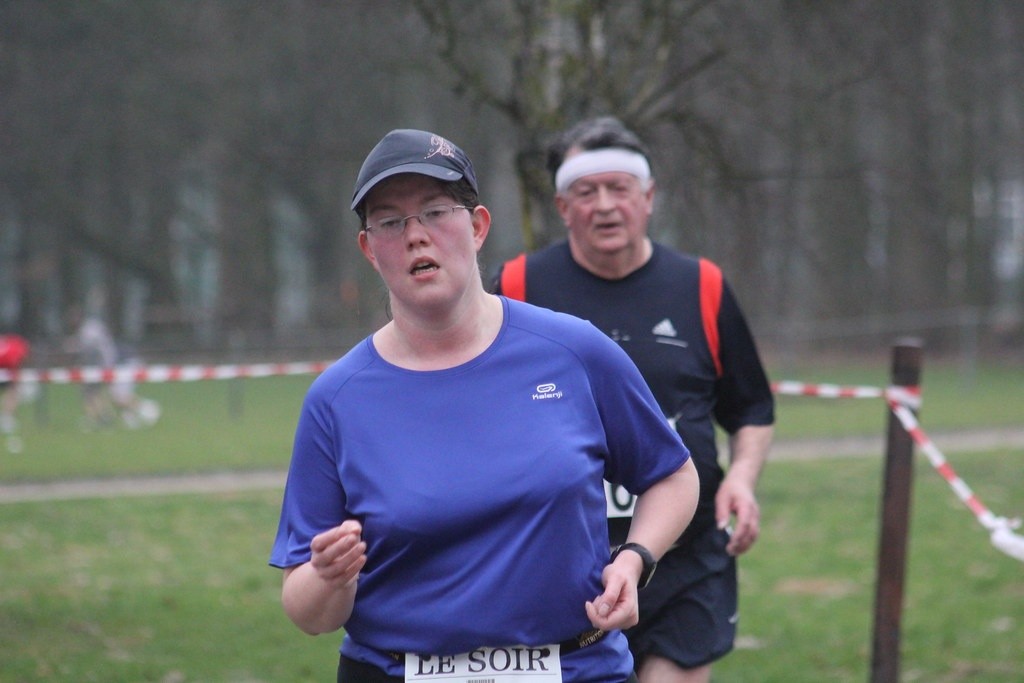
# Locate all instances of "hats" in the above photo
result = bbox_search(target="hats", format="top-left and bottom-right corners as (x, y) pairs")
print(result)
(350, 128), (478, 212)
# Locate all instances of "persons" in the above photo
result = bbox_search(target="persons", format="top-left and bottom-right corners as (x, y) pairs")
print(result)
(486, 115), (778, 683)
(267, 129), (699, 683)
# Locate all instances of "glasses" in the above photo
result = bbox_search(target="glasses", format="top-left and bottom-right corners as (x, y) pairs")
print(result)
(365, 205), (474, 237)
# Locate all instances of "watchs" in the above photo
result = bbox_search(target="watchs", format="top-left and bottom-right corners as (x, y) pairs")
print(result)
(610, 542), (657, 591)
